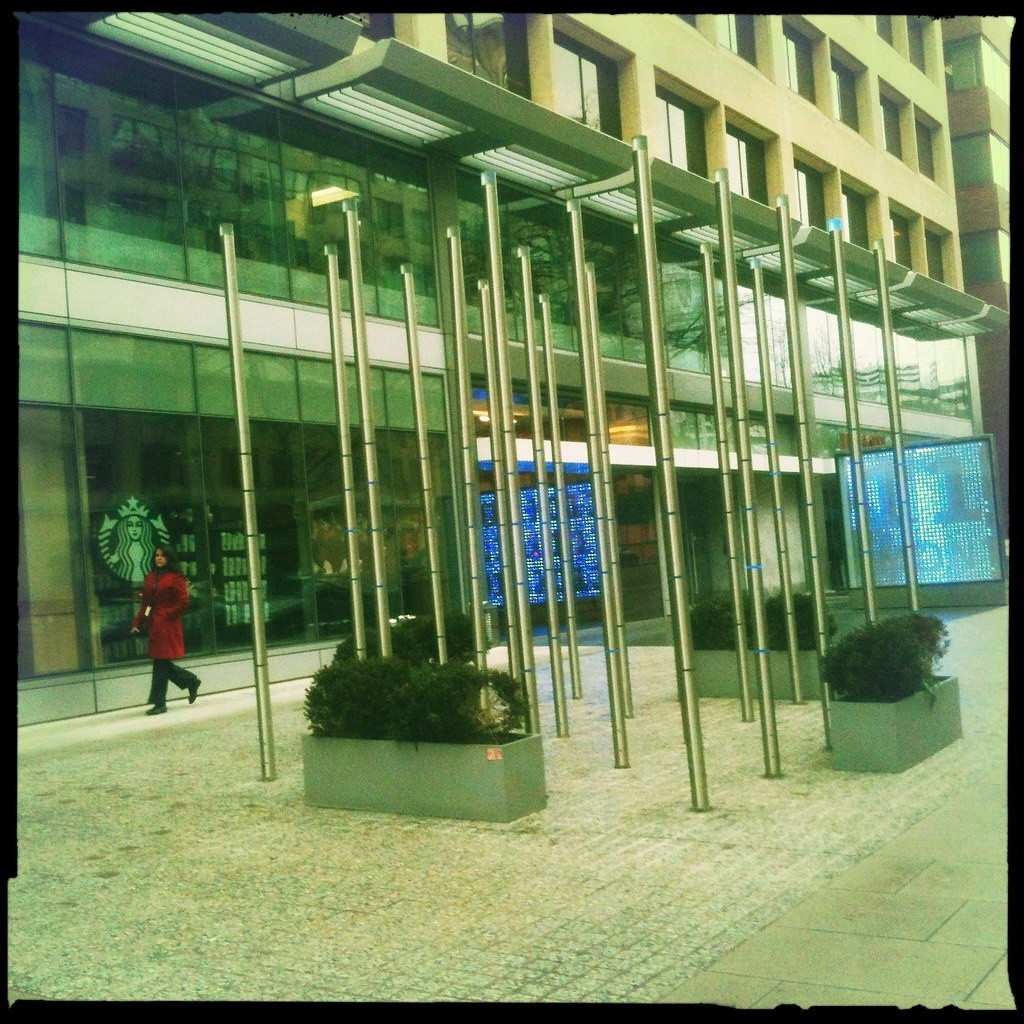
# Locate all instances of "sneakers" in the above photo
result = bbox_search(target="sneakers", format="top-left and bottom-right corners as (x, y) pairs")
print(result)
(144, 705), (168, 716)
(186, 679), (202, 704)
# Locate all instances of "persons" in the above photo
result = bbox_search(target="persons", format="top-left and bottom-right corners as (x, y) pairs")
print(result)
(130, 545), (201, 715)
